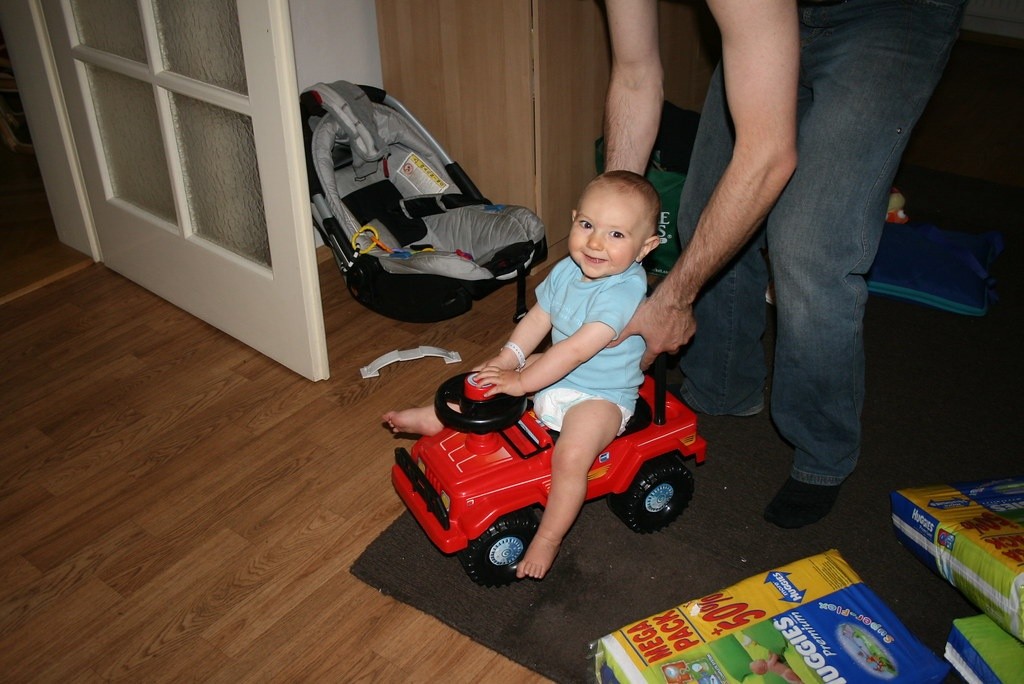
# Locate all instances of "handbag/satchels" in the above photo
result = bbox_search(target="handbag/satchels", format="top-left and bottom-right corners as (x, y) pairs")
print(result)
(866, 222), (1006, 316)
(595, 100), (701, 277)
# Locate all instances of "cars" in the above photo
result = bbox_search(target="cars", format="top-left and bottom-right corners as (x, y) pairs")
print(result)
(389, 349), (708, 588)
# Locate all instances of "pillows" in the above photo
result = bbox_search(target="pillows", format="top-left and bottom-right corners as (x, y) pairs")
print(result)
(869, 225), (1006, 318)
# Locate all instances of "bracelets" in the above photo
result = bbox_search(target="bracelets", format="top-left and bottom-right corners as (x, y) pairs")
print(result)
(503, 342), (525, 369)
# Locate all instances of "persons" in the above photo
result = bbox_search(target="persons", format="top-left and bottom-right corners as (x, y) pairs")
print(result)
(604, 1), (963, 529)
(381, 170), (660, 579)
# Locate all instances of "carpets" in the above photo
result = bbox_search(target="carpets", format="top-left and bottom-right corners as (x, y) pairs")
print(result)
(346, 100), (1024, 684)
